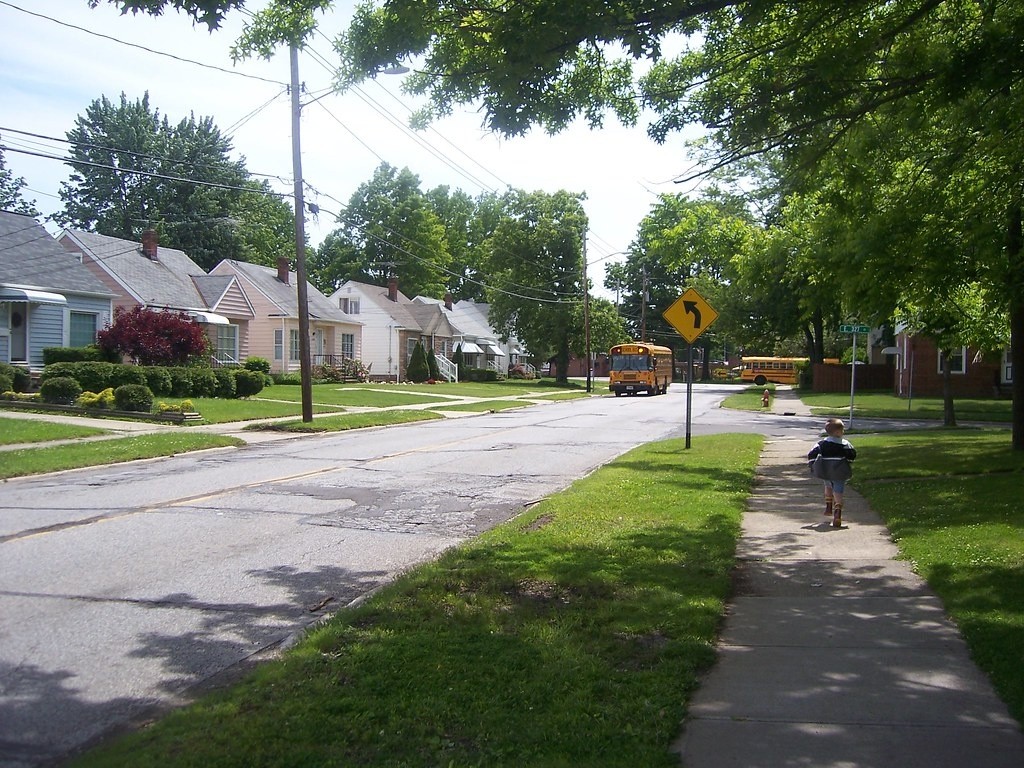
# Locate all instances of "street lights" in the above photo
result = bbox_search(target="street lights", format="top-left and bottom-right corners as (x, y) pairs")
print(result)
(581, 251), (628, 394)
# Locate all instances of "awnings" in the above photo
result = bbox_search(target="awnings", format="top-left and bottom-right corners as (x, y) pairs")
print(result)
(452, 342), (484, 354)
(188, 312), (230, 324)
(510, 349), (520, 355)
(486, 346), (505, 356)
(0, 288), (67, 305)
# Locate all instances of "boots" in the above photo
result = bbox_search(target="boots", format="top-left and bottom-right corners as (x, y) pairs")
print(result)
(824, 497), (833, 516)
(833, 503), (842, 527)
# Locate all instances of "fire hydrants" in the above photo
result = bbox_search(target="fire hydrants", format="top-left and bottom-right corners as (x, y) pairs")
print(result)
(760, 389), (770, 407)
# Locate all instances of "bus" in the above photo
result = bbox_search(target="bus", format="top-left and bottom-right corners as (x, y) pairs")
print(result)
(739, 355), (842, 386)
(607, 340), (673, 396)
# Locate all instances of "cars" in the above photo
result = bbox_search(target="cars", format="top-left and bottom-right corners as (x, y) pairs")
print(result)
(731, 365), (746, 376)
(714, 368), (727, 379)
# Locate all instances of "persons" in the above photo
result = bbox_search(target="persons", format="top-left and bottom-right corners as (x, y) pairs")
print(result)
(808, 419), (856, 529)
